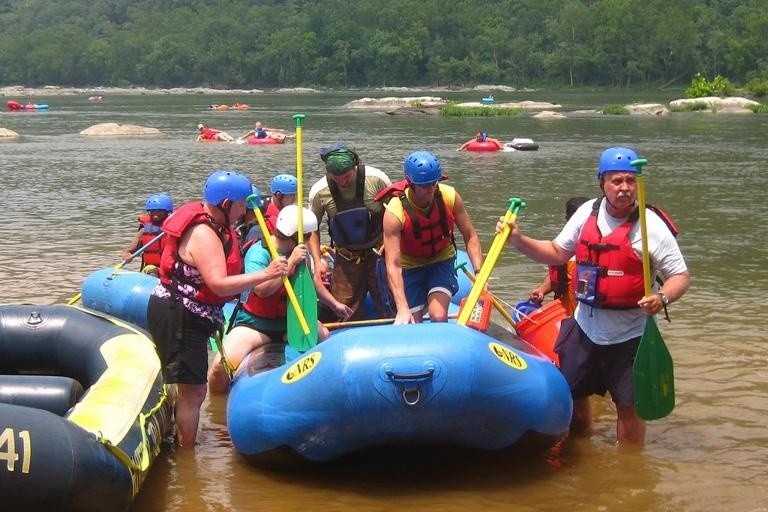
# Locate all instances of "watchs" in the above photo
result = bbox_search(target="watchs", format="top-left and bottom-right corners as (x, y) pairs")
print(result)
(657, 292), (670, 307)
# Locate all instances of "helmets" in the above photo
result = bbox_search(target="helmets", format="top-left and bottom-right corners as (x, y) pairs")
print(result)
(276, 203), (321, 238)
(403, 149), (444, 185)
(204, 170), (254, 206)
(269, 174), (300, 195)
(145, 192), (174, 213)
(597, 145), (643, 178)
(246, 182), (263, 209)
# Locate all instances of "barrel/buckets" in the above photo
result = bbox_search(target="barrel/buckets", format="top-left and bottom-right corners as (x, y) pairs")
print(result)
(516, 299), (569, 370)
(511, 298), (543, 324)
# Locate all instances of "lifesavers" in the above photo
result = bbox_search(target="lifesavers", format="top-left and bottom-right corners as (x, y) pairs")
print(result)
(246, 135), (282, 143)
(466, 142), (498, 152)
(507, 143), (540, 150)
(7, 101), (49, 109)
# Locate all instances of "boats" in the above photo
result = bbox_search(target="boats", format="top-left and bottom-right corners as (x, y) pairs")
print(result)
(80, 267), (161, 340)
(0, 303), (169, 510)
(227, 247), (573, 474)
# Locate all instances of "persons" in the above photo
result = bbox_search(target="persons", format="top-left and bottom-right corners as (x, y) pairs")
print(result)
(121, 193), (172, 277)
(196, 124), (234, 142)
(494, 146), (690, 445)
(158, 171), (288, 449)
(207, 142), (593, 394)
(455, 132), (503, 152)
(240, 121), (286, 143)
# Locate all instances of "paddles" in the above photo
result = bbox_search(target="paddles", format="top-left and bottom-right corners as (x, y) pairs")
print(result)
(632, 159), (674, 420)
(287, 114), (317, 352)
(66, 233), (167, 306)
